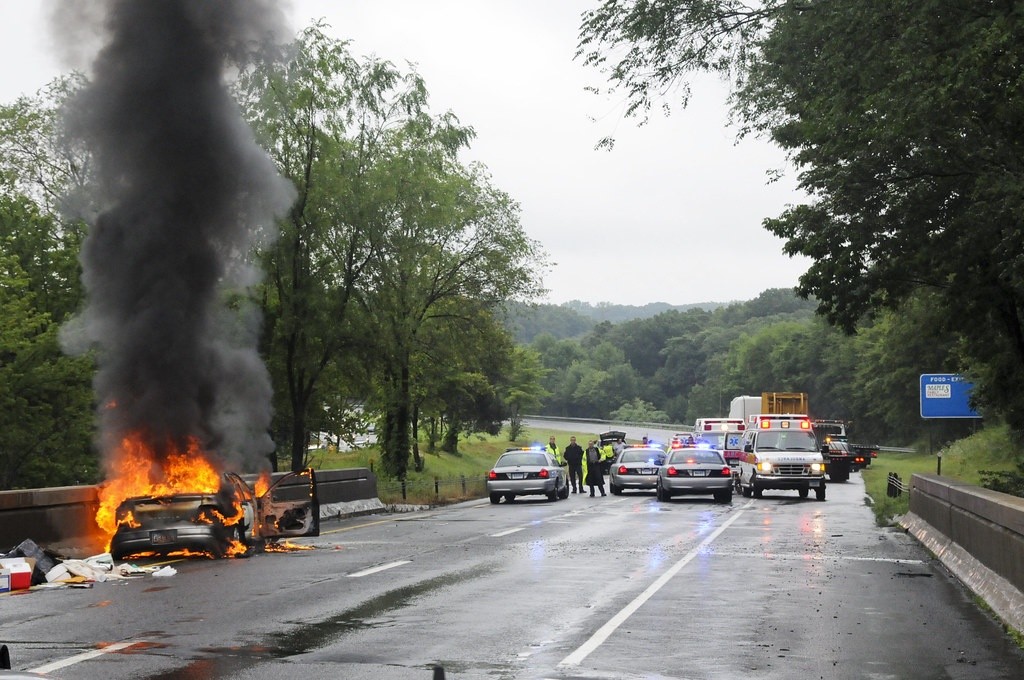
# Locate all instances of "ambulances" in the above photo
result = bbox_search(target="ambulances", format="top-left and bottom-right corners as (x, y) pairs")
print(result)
(670, 433), (697, 449)
(690, 417), (746, 484)
(734, 413), (831, 503)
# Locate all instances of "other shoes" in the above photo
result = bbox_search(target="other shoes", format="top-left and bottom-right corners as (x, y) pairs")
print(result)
(580, 489), (587, 493)
(602, 492), (606, 496)
(591, 493), (594, 497)
(571, 489), (576, 493)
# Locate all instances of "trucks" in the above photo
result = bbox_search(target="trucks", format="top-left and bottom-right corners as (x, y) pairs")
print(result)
(812, 418), (851, 483)
(847, 440), (880, 472)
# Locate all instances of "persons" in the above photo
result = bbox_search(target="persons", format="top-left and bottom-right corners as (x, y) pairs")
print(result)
(582, 440), (606, 496)
(687, 437), (695, 444)
(594, 439), (625, 475)
(564, 436), (586, 493)
(642, 437), (647, 444)
(546, 436), (561, 465)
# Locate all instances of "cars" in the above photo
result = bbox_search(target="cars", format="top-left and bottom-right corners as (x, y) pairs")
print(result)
(486, 446), (570, 502)
(592, 430), (627, 475)
(609, 443), (668, 496)
(108, 466), (322, 565)
(656, 443), (735, 504)
